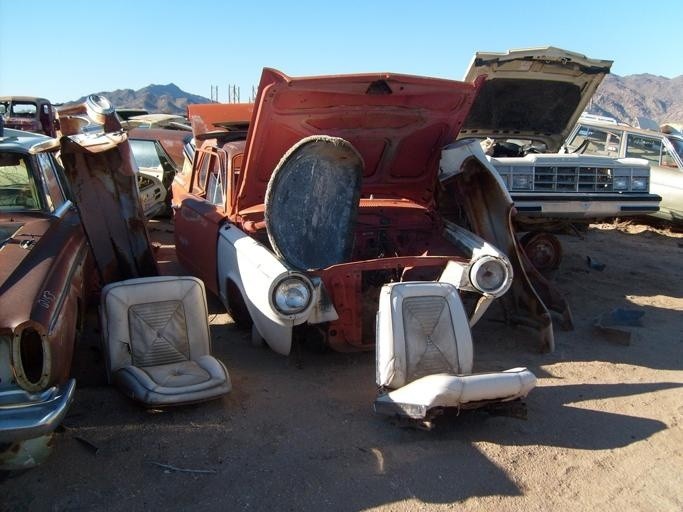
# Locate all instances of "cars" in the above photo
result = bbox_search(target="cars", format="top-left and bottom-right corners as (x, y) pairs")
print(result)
(174, 67), (515, 356)
(0, 128), (90, 444)
(464, 46), (662, 225)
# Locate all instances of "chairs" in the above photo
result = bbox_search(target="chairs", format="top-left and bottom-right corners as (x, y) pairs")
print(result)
(374, 280), (538, 433)
(95, 276), (232, 409)
